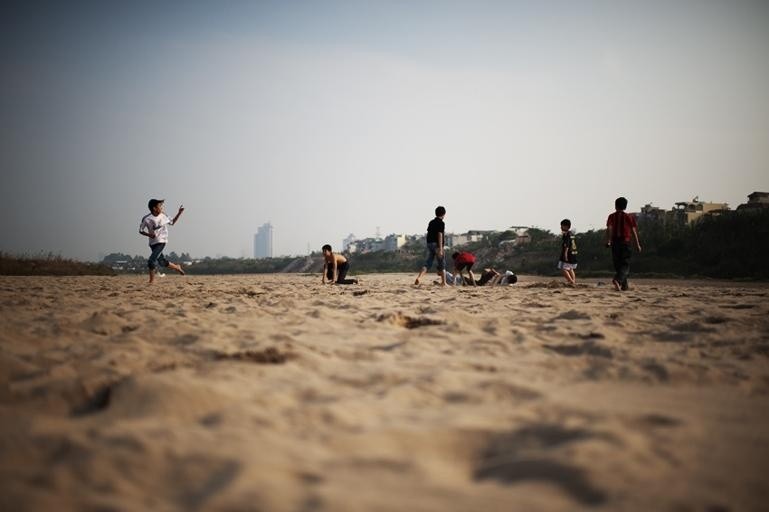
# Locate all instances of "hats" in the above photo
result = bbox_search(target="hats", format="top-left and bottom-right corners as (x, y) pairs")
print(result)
(147, 198), (164, 208)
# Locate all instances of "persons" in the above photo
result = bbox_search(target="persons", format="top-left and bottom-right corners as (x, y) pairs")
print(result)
(415, 206), (452, 288)
(433, 268), (497, 286)
(557, 219), (578, 284)
(139, 199), (185, 282)
(606, 197), (642, 290)
(452, 252), (477, 286)
(482, 269), (517, 286)
(322, 245), (358, 285)
